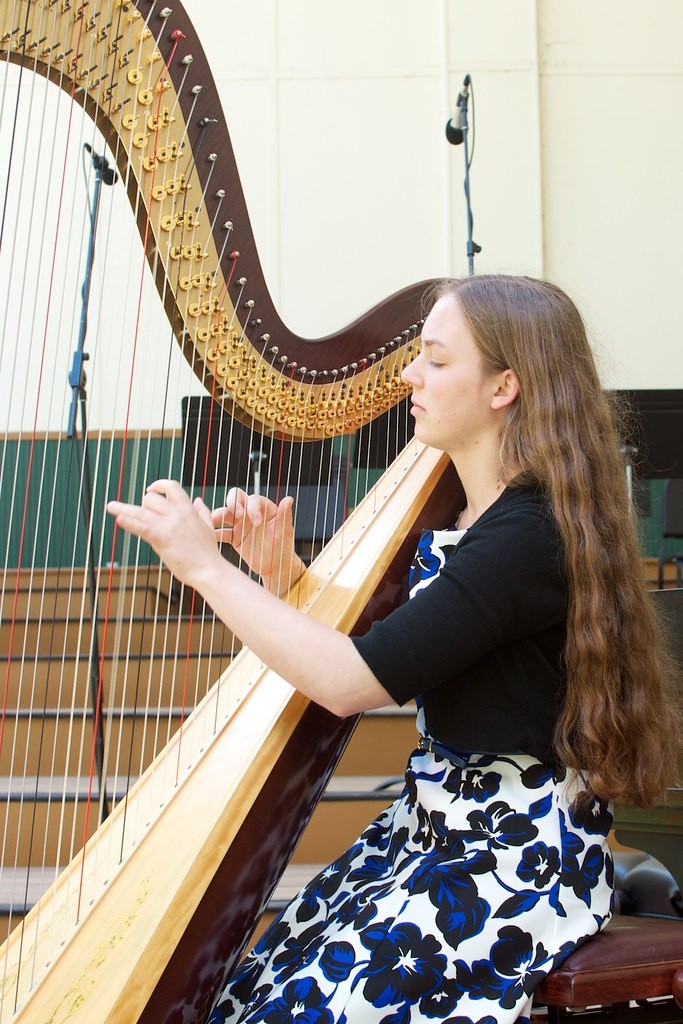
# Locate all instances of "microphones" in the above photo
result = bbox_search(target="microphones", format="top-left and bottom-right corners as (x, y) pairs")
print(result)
(446, 75), (471, 144)
(85, 144), (119, 186)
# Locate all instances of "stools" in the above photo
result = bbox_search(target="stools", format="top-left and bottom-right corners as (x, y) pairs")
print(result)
(534, 914), (683, 1024)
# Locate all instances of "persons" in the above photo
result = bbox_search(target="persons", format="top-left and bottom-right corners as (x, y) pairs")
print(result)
(105, 276), (683, 1024)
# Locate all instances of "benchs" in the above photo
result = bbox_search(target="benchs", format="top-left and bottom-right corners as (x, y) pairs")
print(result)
(0, 565), (419, 968)
